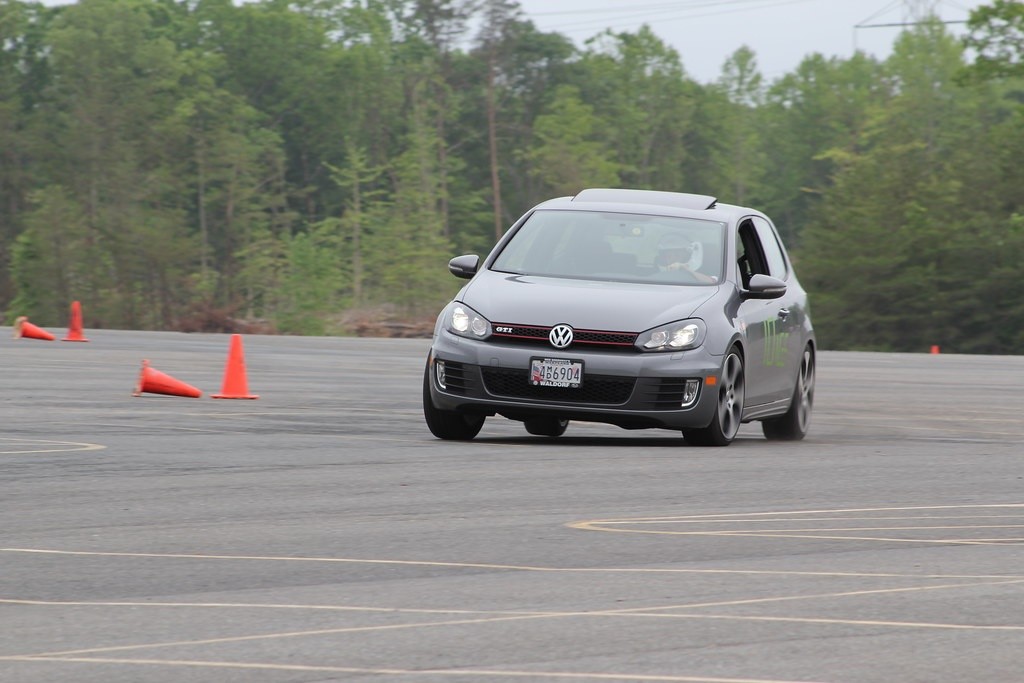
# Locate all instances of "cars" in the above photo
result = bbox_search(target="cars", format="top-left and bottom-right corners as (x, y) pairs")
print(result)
(422, 188), (818, 447)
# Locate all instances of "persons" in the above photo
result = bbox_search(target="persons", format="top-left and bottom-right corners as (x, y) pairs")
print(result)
(651, 231), (720, 284)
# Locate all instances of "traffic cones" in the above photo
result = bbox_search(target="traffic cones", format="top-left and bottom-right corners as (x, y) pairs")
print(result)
(134, 359), (202, 398)
(13, 316), (56, 341)
(210, 334), (259, 398)
(58, 301), (89, 342)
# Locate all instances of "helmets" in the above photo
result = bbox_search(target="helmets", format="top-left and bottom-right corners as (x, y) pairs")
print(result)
(656, 233), (703, 271)
